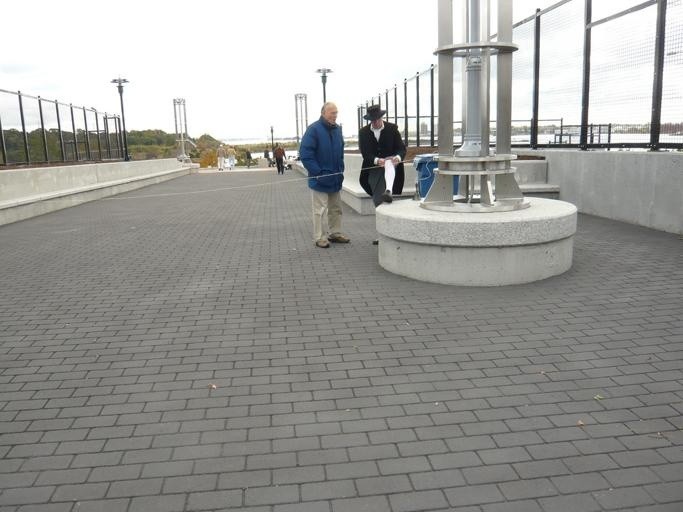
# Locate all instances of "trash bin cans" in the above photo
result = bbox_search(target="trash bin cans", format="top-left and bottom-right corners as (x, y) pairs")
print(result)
(413, 154), (459, 197)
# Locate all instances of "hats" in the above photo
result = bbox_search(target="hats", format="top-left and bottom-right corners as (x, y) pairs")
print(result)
(364, 105), (387, 119)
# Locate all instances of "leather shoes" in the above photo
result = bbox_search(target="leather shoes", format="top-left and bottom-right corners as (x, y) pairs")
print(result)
(316, 238), (330, 248)
(382, 189), (393, 202)
(328, 235), (350, 243)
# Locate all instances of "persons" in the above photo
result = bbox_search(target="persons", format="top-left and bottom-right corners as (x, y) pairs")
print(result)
(216, 144), (226, 171)
(246, 149), (258, 168)
(263, 143), (286, 175)
(226, 144), (236, 170)
(359, 105), (406, 245)
(299, 102), (349, 248)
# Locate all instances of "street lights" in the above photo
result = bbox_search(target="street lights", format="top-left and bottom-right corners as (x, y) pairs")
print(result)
(110, 77), (131, 161)
(316, 68), (333, 104)
